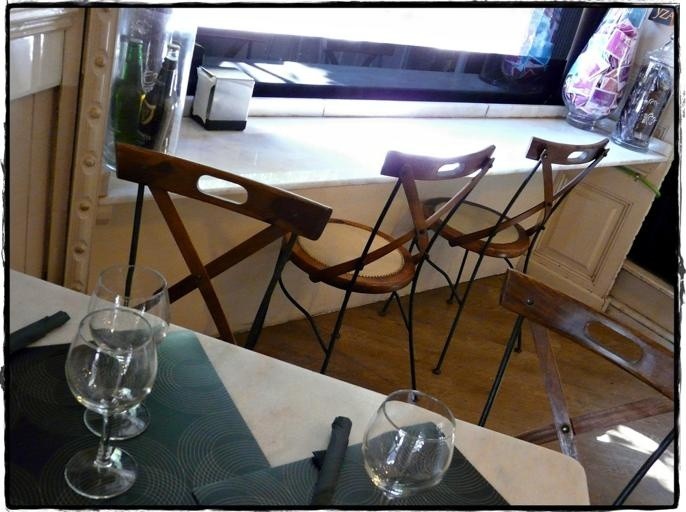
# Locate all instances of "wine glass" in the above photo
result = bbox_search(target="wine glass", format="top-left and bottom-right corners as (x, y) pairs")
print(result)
(361, 388), (457, 509)
(83, 265), (172, 441)
(63, 305), (158, 501)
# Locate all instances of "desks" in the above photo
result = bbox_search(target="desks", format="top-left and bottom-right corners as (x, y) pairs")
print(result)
(91, 117), (674, 314)
(10, 267), (590, 511)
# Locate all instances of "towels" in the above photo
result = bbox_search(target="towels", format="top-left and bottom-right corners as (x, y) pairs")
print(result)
(313, 416), (352, 504)
(9, 310), (70, 353)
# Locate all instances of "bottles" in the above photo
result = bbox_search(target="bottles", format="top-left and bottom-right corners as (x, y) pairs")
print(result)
(133, 42), (181, 154)
(102, 39), (143, 166)
(612, 32), (674, 154)
